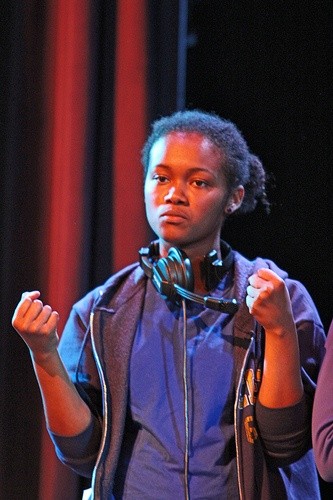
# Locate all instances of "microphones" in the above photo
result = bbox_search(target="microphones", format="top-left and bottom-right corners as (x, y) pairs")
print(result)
(173, 285), (239, 315)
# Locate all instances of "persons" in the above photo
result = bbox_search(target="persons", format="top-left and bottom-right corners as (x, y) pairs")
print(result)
(11, 111), (326, 500)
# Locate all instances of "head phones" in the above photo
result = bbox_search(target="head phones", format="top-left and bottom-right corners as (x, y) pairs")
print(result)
(139, 238), (235, 302)
